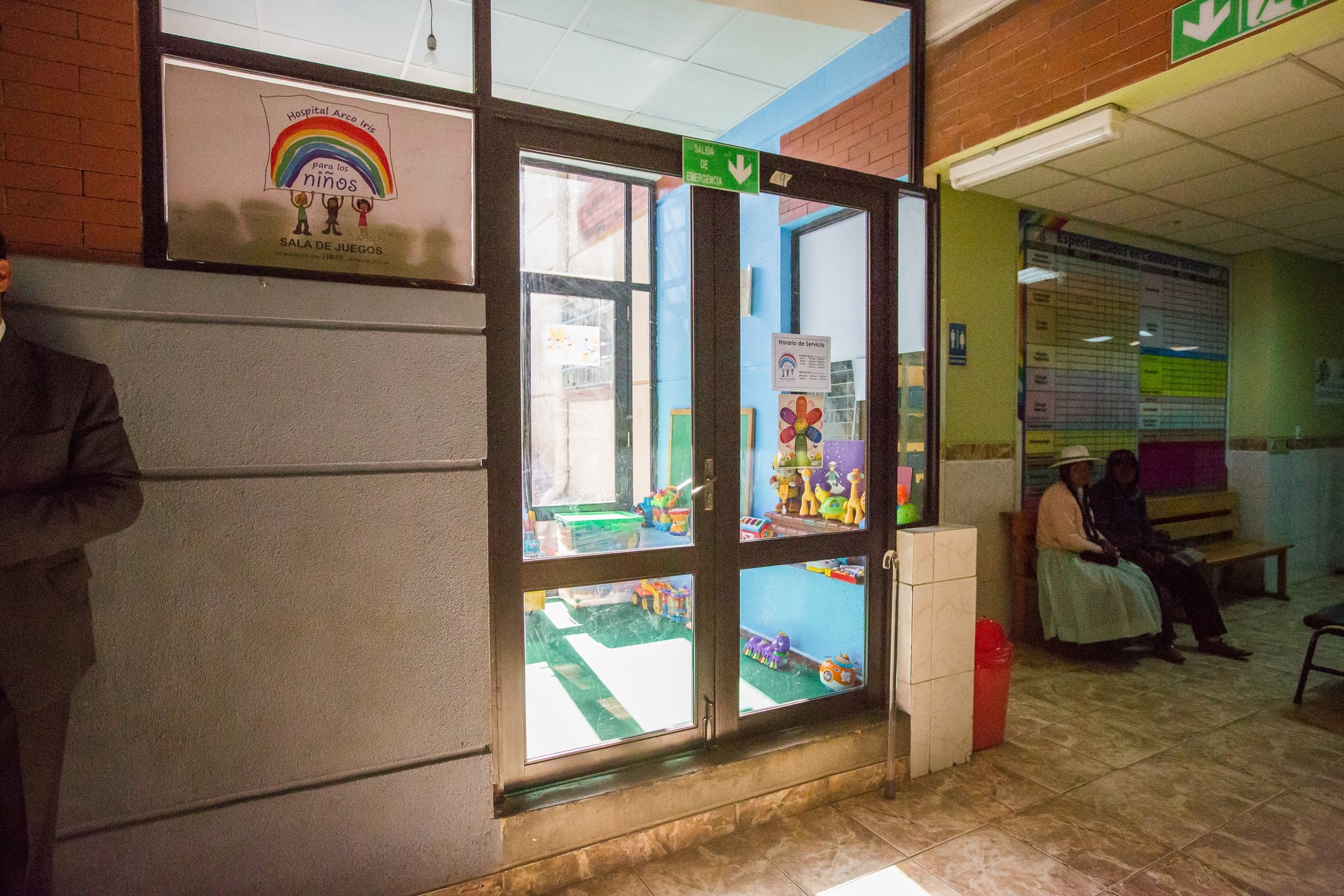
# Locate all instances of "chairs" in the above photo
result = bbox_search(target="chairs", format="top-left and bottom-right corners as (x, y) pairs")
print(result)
(1292, 601), (1344, 704)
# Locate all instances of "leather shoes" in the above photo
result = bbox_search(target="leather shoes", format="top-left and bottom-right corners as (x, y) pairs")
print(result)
(1198, 637), (1254, 656)
(1151, 642), (1186, 663)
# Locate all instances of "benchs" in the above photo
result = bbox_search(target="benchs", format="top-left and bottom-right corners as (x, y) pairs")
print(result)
(1008, 490), (1296, 639)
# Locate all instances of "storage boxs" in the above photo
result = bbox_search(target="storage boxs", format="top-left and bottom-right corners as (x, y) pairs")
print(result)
(554, 552), (647, 609)
(554, 511), (644, 554)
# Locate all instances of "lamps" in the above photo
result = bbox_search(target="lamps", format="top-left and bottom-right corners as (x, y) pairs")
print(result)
(950, 102), (1128, 191)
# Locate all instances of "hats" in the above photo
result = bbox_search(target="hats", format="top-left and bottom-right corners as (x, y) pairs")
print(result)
(1048, 445), (1099, 468)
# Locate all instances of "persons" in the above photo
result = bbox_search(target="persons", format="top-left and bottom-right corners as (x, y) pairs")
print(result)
(1085, 449), (1255, 662)
(1034, 445), (1162, 644)
(0, 238), (142, 896)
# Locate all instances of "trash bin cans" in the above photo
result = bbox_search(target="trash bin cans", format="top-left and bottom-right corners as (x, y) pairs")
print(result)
(971, 612), (1012, 751)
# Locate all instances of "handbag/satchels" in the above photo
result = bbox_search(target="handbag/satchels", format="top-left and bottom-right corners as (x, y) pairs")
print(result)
(1080, 535), (1118, 566)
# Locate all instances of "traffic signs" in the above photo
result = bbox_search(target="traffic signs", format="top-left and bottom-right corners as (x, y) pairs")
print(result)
(1170, 0), (1322, 63)
(682, 134), (761, 194)
